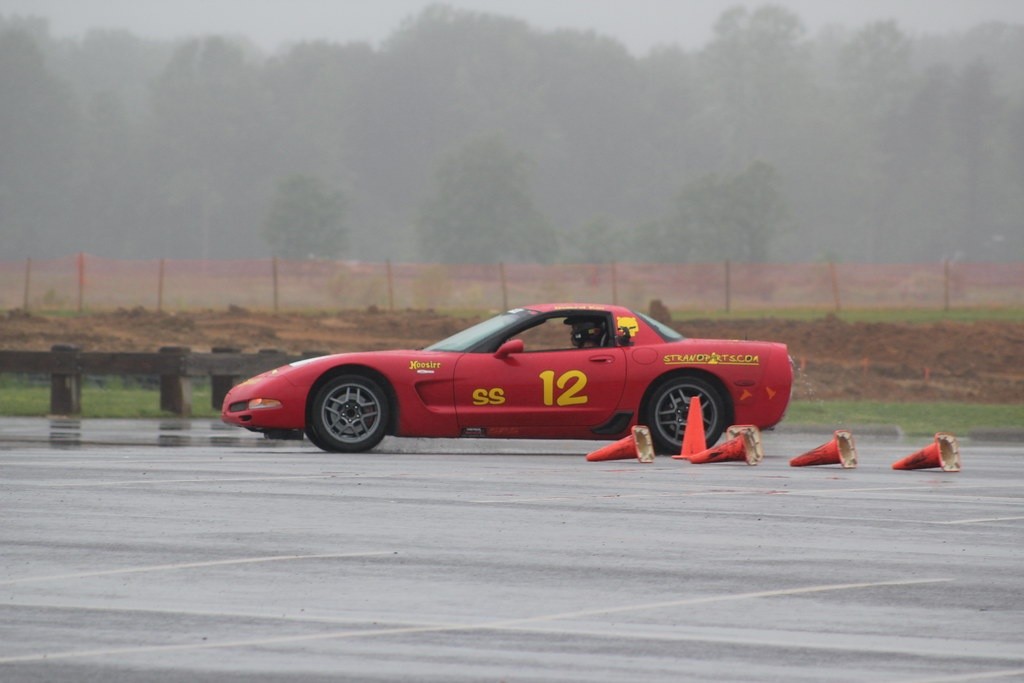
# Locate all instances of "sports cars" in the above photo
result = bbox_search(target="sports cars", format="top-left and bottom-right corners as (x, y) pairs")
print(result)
(222, 304), (794, 453)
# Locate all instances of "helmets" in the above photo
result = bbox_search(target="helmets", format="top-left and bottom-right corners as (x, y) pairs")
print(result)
(563, 316), (607, 343)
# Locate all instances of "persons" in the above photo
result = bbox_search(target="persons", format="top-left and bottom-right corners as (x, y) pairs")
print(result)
(563, 316), (602, 349)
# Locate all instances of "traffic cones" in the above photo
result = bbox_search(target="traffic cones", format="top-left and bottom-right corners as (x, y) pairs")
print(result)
(669, 398), (707, 460)
(893, 429), (961, 473)
(789, 430), (858, 468)
(726, 424), (763, 464)
(689, 429), (763, 466)
(585, 426), (655, 463)
